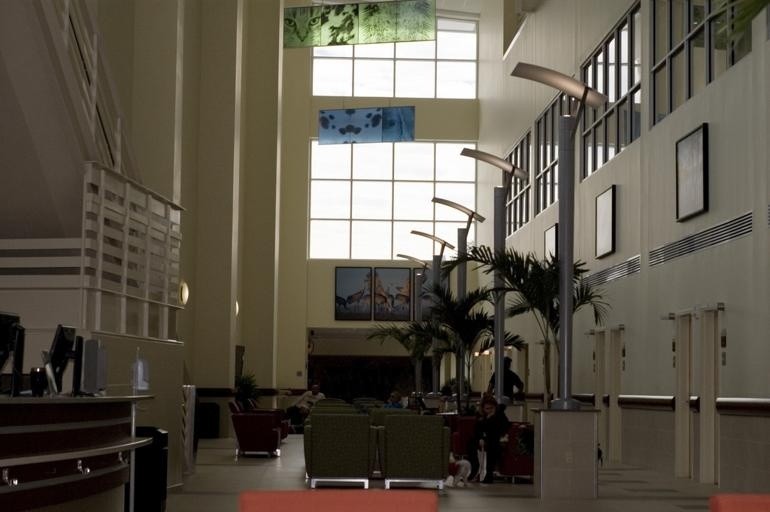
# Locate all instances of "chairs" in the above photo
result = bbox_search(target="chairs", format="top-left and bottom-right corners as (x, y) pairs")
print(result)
(228, 399), (288, 461)
(453, 415), (536, 483)
(302, 398), (453, 489)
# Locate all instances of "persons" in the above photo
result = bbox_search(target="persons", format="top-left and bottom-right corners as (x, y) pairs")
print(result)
(467, 395), (513, 483)
(487, 356), (524, 399)
(291, 384), (326, 419)
(382, 391), (403, 408)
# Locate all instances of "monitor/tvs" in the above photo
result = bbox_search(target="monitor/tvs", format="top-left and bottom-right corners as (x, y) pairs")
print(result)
(45, 323), (85, 396)
(0, 312), (25, 396)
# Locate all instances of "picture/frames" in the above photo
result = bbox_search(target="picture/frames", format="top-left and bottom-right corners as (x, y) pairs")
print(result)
(413, 267), (451, 322)
(335, 266), (373, 322)
(374, 267), (411, 323)
(545, 224), (559, 268)
(595, 186), (615, 260)
(675, 122), (710, 222)
